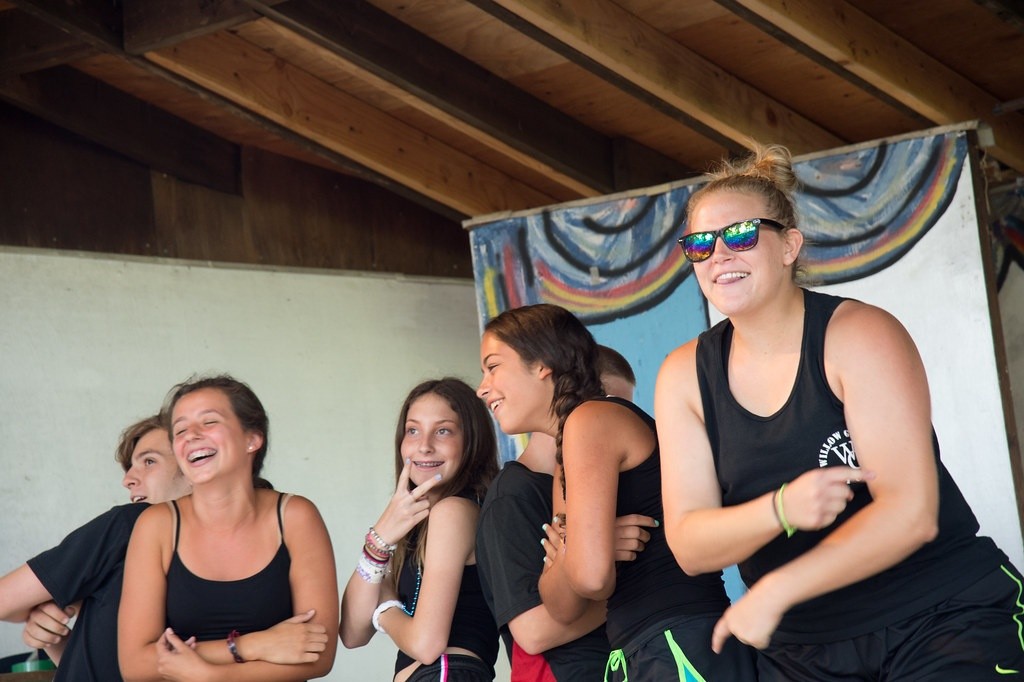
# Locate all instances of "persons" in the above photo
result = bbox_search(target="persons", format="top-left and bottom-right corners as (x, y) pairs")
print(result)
(115, 374), (339, 682)
(475, 303), (754, 682)
(480, 345), (637, 681)
(654, 145), (1024, 682)
(0, 410), (193, 682)
(333, 376), (499, 681)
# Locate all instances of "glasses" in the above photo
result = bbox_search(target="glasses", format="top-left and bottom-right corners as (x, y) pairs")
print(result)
(677, 217), (788, 261)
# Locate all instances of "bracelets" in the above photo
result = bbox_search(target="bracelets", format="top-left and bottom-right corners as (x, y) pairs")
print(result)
(354, 524), (398, 585)
(226, 629), (244, 664)
(370, 599), (404, 635)
(770, 482), (795, 537)
